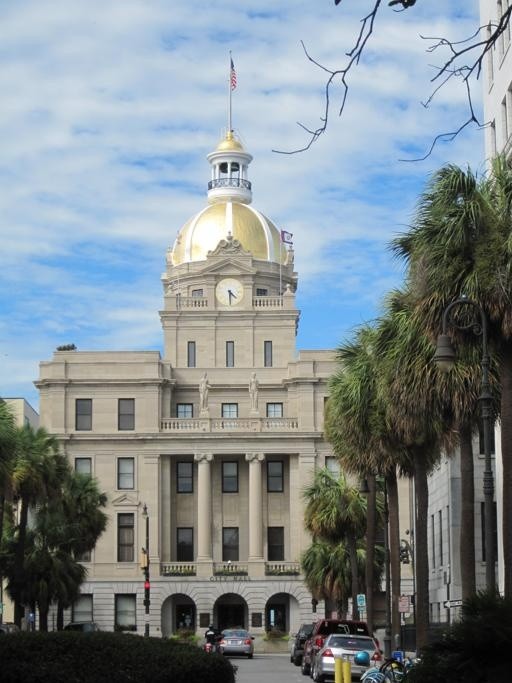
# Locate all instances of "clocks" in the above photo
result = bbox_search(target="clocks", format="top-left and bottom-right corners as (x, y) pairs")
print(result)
(215, 278), (244, 306)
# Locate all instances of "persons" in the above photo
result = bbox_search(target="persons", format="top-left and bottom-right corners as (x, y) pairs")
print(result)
(205, 625), (221, 654)
(249, 372), (259, 409)
(199, 371), (211, 410)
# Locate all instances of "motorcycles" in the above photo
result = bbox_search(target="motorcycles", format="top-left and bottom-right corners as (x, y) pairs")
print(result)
(203, 634), (225, 656)
(354, 651), (421, 683)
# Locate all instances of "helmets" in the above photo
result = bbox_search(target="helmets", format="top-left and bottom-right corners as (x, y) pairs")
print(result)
(209, 624), (214, 627)
(355, 652), (370, 666)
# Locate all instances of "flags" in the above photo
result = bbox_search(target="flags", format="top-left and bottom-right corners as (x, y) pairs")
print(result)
(281, 231), (294, 245)
(231, 54), (237, 91)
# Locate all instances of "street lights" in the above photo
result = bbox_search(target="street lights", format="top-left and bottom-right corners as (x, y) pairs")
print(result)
(434, 294), (495, 590)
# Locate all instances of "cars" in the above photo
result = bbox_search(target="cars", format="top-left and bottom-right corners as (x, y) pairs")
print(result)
(63, 621), (100, 632)
(291, 619), (385, 682)
(220, 629), (255, 659)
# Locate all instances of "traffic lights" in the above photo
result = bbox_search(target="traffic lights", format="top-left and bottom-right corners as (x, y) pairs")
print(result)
(145, 580), (150, 597)
(141, 567), (147, 577)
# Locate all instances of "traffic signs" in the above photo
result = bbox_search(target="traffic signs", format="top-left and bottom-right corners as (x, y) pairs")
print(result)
(444, 600), (462, 608)
